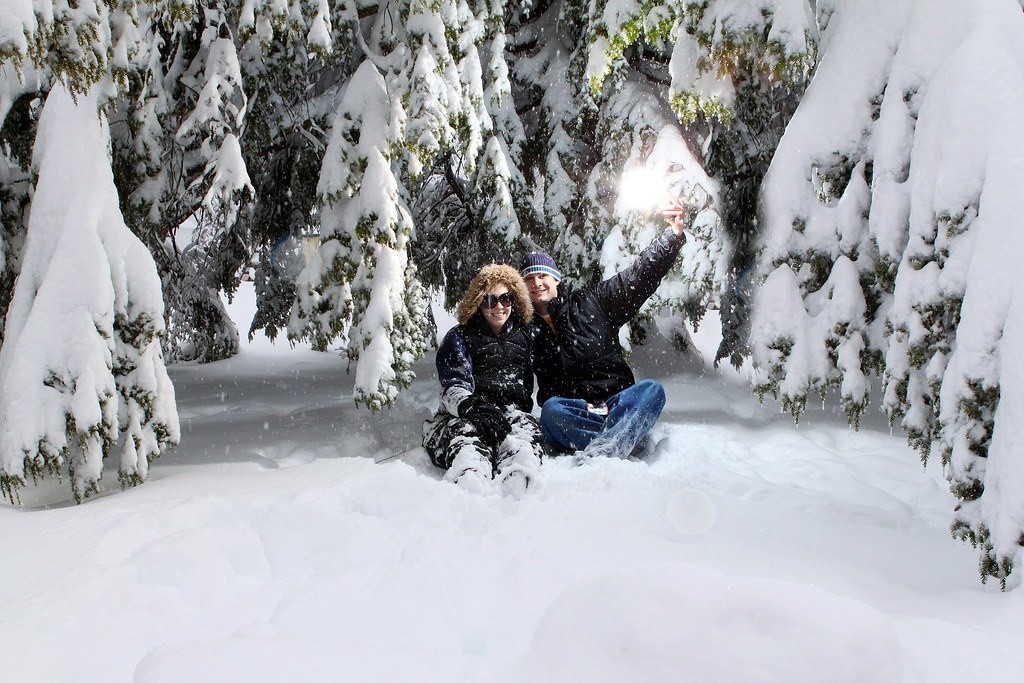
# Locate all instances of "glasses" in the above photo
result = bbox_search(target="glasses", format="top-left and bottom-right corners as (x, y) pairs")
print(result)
(480, 292), (514, 309)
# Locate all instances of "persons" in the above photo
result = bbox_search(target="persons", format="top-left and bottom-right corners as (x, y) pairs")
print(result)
(421, 261), (547, 497)
(516, 197), (687, 458)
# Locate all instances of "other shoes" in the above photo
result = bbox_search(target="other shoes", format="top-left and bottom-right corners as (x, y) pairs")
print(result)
(454, 468), (491, 485)
(501, 470), (529, 494)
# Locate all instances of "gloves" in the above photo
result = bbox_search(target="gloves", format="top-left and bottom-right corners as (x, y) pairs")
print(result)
(540, 376), (576, 403)
(457, 393), (512, 446)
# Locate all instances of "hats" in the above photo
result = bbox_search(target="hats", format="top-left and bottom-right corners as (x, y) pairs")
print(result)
(519, 251), (561, 282)
